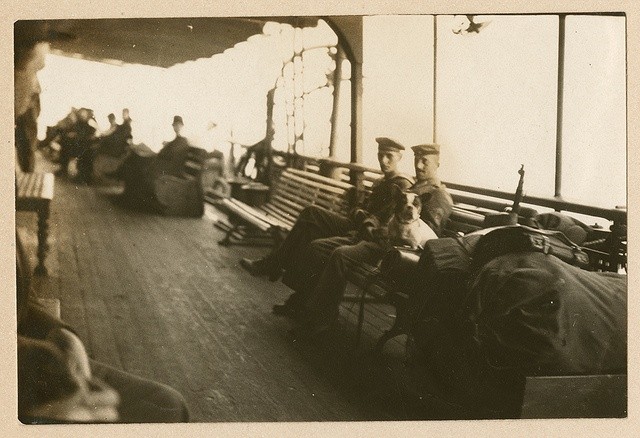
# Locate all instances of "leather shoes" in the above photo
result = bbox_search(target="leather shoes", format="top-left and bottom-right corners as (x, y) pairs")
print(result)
(239, 254), (284, 283)
(272, 303), (291, 314)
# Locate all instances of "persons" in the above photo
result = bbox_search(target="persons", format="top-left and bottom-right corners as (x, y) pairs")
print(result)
(34, 106), (132, 183)
(14, 28), (190, 424)
(102, 115), (190, 201)
(239, 137), (415, 314)
(272, 143), (454, 338)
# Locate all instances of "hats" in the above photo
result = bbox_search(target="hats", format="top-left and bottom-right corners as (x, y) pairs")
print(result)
(172, 115), (183, 126)
(375, 138), (406, 152)
(411, 144), (440, 156)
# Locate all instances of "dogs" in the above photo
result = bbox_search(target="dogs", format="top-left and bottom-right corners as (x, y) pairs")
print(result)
(386, 184), (440, 254)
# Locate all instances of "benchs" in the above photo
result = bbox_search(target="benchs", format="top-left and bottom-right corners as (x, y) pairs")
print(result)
(219, 167), (355, 247)
(305, 192), (549, 387)
(15, 144), (55, 274)
(179, 146), (207, 186)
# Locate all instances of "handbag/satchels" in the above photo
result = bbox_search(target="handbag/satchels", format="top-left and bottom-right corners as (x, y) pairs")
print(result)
(470, 226), (590, 268)
